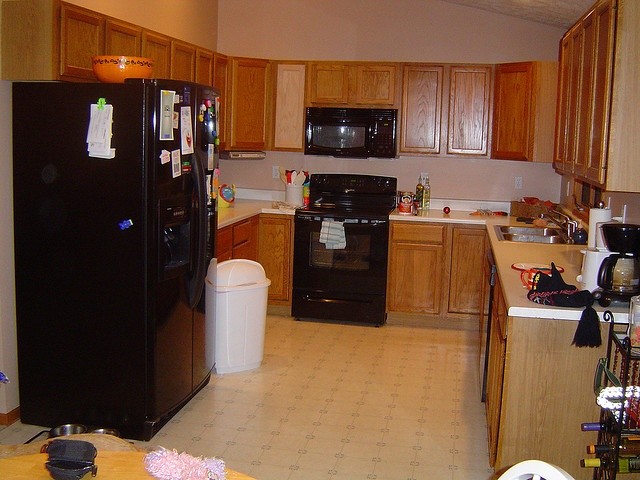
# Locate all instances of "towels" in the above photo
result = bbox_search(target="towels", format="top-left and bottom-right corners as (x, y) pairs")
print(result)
(319, 219), (347, 250)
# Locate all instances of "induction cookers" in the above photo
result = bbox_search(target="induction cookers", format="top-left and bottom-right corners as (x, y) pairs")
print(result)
(295, 174), (398, 223)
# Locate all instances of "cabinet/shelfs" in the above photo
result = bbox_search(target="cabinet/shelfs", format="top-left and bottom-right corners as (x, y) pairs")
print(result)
(481, 273), (639, 480)
(143, 27), (195, 83)
(555, 17), (581, 178)
(195, 47), (229, 151)
(495, 61), (560, 163)
(592, 310), (640, 479)
(575, 0), (640, 193)
(448, 219), (488, 314)
(1, 0), (142, 84)
(215, 210), (259, 263)
(389, 216), (447, 317)
(397, 62), (494, 160)
(257, 209), (294, 305)
(305, 63), (399, 110)
(269, 58), (309, 152)
(228, 56), (269, 151)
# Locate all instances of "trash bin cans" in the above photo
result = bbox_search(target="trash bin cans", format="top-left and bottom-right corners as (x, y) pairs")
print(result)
(204, 259), (271, 375)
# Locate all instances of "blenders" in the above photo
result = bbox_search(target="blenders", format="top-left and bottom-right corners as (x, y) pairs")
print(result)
(9, 78), (218, 442)
(576, 196), (629, 293)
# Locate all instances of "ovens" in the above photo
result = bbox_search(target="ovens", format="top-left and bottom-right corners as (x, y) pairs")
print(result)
(291, 217), (397, 328)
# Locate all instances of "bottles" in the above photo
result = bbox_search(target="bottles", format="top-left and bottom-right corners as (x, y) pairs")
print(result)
(423, 175), (431, 210)
(581, 420), (640, 437)
(586, 441), (640, 457)
(581, 456), (640, 474)
(416, 178), (424, 209)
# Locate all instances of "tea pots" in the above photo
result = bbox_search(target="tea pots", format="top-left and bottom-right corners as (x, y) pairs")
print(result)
(395, 194), (414, 214)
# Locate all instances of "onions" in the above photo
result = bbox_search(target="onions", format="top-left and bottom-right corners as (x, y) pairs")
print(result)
(443, 207), (450, 214)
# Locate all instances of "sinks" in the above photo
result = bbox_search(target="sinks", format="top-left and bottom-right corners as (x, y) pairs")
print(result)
(494, 225), (565, 236)
(497, 234), (566, 244)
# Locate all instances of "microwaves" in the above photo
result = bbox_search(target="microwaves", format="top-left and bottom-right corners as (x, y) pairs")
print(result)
(304, 106), (397, 159)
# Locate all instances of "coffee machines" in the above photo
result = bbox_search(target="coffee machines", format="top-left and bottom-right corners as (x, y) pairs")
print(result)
(597, 223), (640, 307)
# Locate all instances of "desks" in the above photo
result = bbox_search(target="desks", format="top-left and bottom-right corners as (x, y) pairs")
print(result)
(0, 431), (254, 480)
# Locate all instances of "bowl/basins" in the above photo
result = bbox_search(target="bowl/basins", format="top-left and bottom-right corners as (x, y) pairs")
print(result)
(91, 55), (154, 85)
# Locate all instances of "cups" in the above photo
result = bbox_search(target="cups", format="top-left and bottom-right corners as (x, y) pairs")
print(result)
(285, 184), (305, 208)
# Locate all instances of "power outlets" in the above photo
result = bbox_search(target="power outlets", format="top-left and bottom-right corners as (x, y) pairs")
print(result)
(272, 165), (280, 179)
(514, 176), (522, 190)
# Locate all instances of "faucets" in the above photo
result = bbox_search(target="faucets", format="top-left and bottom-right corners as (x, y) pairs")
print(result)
(538, 209), (577, 237)
